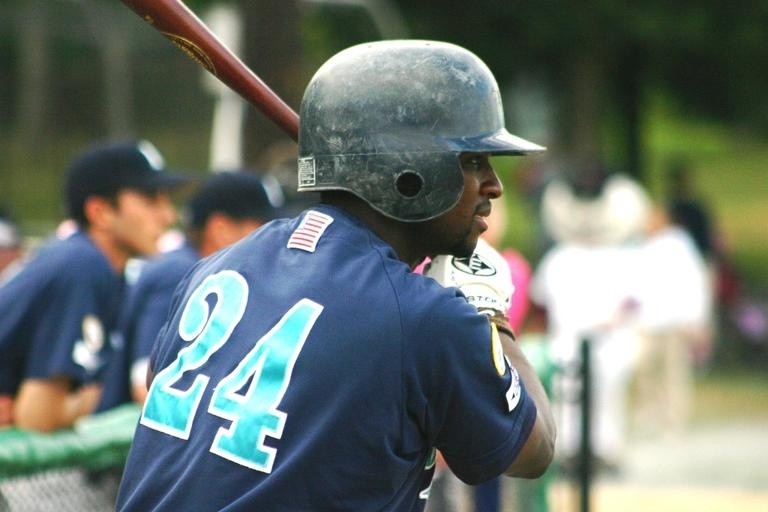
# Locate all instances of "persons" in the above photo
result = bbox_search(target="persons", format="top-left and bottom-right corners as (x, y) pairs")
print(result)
(433, 141), (722, 512)
(1, 139), (308, 511)
(110, 38), (559, 510)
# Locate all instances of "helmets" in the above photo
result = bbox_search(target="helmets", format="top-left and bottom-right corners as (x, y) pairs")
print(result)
(295, 38), (549, 224)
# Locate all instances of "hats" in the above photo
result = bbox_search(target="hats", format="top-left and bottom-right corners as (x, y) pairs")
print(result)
(65, 142), (189, 216)
(188, 170), (292, 225)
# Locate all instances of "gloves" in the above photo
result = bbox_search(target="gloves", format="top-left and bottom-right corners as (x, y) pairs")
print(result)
(421, 230), (517, 342)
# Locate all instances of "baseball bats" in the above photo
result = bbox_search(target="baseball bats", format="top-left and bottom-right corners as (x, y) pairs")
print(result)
(123, 0), (300, 143)
